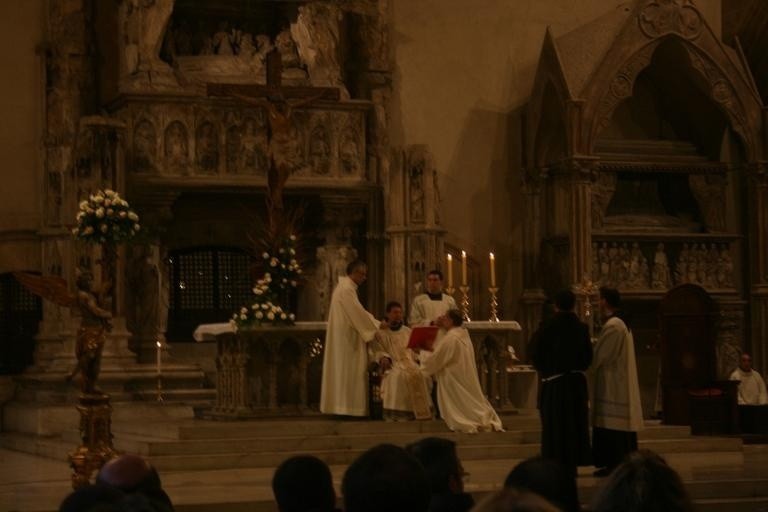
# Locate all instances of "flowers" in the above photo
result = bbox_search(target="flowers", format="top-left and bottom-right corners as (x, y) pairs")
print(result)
(73, 189), (140, 243)
(231, 235), (303, 325)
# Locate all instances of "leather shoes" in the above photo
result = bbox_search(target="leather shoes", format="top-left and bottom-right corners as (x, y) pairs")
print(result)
(593, 467), (612, 476)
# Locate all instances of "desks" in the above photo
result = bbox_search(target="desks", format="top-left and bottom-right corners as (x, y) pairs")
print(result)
(193, 319), (522, 421)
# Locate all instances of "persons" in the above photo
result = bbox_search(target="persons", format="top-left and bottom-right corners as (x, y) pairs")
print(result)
(729, 354), (767, 405)
(583, 287), (645, 478)
(228, 84), (326, 214)
(589, 240), (736, 289)
(322, 260), (388, 416)
(71, 265), (116, 395)
(525, 289), (593, 474)
(271, 437), (693, 511)
(407, 270), (458, 392)
(59, 454), (174, 511)
(133, 117), (362, 175)
(412, 308), (505, 432)
(368, 300), (438, 420)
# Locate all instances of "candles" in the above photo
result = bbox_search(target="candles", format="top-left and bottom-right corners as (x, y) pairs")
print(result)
(156, 340), (161, 372)
(461, 250), (467, 286)
(447, 253), (453, 288)
(489, 252), (496, 287)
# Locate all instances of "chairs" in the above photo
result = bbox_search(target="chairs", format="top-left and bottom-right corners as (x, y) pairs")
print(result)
(658, 282), (741, 432)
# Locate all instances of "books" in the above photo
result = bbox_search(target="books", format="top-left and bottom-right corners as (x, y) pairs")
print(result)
(407, 326), (438, 351)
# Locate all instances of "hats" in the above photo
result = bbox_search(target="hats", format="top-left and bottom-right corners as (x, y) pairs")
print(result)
(99, 454), (149, 487)
(504, 455), (580, 512)
(452, 309), (465, 320)
